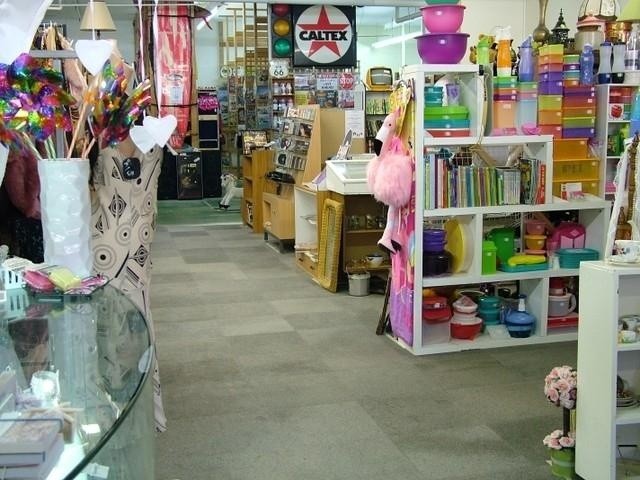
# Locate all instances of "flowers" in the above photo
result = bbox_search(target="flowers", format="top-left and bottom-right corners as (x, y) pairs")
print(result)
(539, 365), (577, 450)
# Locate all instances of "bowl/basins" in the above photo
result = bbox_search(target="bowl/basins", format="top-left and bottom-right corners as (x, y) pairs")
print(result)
(413, 1), (471, 64)
(524, 221), (547, 250)
(421, 295), (535, 339)
(422, 228), (447, 251)
(549, 280), (573, 318)
(424, 250), (455, 280)
(364, 253), (384, 267)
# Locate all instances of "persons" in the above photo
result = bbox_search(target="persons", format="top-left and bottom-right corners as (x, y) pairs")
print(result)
(89, 89), (167, 452)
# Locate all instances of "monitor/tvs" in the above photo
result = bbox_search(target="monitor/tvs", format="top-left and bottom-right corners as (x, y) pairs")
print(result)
(366, 67), (393, 91)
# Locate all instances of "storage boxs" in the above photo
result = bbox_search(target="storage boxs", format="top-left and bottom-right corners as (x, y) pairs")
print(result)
(537, 42), (580, 66)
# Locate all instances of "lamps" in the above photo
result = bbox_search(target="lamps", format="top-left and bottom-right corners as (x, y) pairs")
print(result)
(78, 0), (119, 40)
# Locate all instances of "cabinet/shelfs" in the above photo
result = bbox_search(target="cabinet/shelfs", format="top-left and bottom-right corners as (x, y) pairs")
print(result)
(574, 260), (640, 479)
(0, 265), (160, 480)
(384, 63), (640, 356)
(218, 2), (396, 294)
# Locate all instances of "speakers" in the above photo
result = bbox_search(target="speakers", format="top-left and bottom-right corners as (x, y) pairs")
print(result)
(157, 152), (178, 200)
(201, 150), (221, 198)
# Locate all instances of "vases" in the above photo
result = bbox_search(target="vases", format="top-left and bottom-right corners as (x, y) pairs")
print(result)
(37, 156), (95, 282)
(550, 448), (575, 478)
(46, 302), (100, 410)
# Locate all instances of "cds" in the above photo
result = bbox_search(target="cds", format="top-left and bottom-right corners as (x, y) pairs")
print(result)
(279, 155), (286, 164)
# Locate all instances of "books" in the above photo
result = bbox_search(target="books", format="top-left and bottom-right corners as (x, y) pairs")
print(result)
(0, 414), (65, 480)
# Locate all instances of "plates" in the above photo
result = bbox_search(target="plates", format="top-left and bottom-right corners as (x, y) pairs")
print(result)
(617, 391), (639, 411)
(607, 256), (640, 267)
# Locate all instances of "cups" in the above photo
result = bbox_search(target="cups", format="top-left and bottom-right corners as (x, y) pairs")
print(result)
(619, 313), (640, 341)
(615, 238), (640, 261)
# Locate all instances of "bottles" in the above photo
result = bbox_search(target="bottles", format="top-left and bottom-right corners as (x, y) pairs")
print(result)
(346, 214), (386, 231)
(579, 42), (626, 86)
(626, 26), (640, 70)
(274, 66), (356, 111)
(476, 26), (533, 82)
